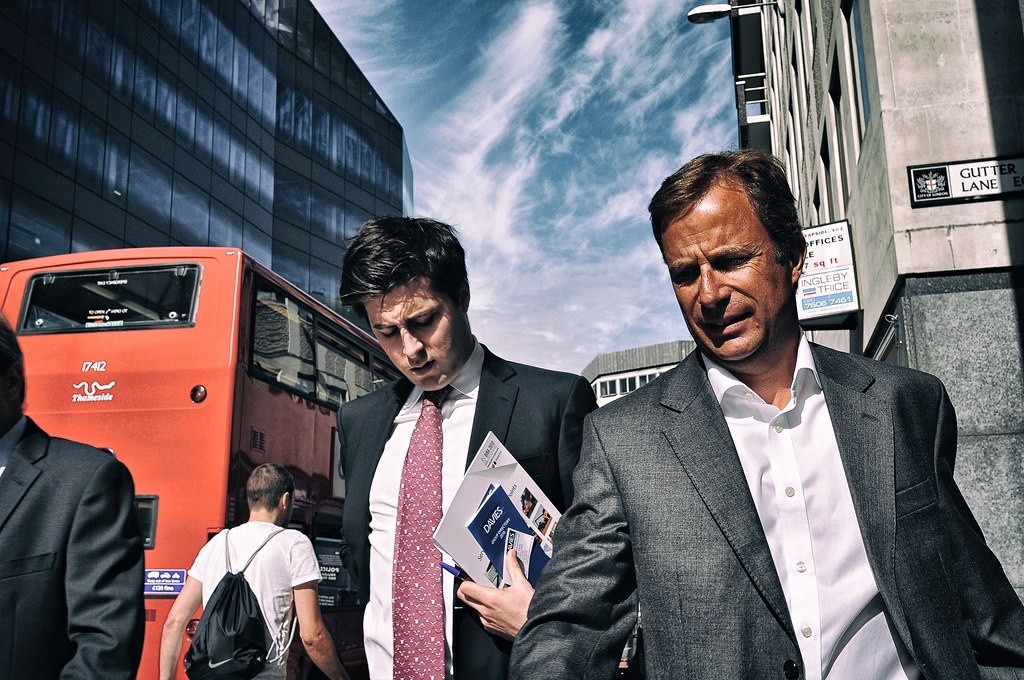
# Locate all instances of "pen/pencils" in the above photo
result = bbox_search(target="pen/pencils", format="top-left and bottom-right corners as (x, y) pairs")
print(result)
(439, 562), (474, 583)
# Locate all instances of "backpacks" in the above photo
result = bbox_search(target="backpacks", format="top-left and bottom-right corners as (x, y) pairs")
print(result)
(183, 527), (284, 680)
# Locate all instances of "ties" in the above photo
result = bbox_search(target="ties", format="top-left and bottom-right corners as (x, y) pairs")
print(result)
(392, 385), (455, 680)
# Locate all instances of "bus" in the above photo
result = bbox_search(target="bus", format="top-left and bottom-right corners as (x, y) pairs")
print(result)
(0, 235), (413, 680)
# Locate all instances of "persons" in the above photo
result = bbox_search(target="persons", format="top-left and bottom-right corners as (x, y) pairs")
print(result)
(0, 312), (145, 680)
(159, 462), (351, 680)
(505, 146), (1024, 679)
(337, 213), (602, 680)
